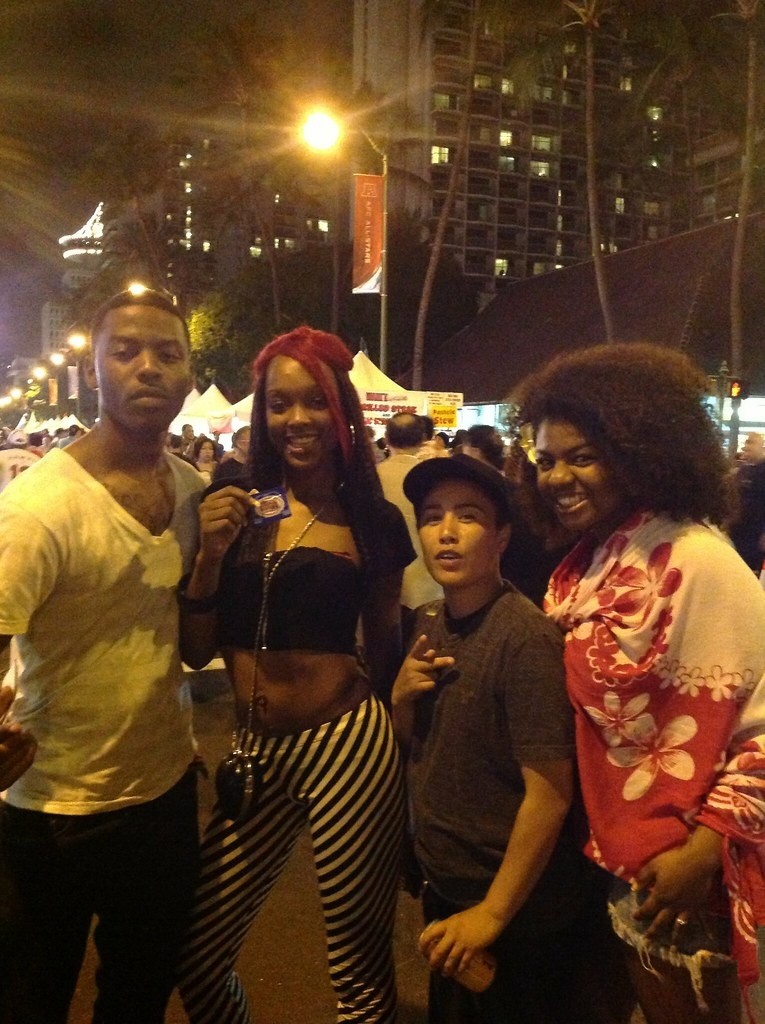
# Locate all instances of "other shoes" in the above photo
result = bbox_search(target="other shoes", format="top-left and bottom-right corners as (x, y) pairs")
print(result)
(192, 679), (231, 703)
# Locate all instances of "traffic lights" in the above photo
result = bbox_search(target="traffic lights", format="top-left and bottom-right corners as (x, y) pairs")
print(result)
(729, 379), (748, 399)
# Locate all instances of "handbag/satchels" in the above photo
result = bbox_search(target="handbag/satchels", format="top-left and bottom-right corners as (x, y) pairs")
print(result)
(214, 751), (262, 823)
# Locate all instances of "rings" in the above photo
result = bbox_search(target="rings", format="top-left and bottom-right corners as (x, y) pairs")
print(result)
(675, 916), (688, 926)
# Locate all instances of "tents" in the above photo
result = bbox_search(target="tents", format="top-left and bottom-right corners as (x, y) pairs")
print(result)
(13, 411), (90, 437)
(167, 348), (426, 444)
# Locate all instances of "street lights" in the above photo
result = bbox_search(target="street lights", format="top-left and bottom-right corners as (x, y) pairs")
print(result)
(297, 107), (348, 335)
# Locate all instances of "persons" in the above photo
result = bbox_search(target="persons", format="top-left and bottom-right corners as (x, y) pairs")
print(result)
(164, 423), (253, 482)
(175, 326), (418, 1024)
(390, 454), (604, 1023)
(702, 430), (765, 584)
(0, 425), (86, 493)
(0, 292), (213, 1024)
(366, 414), (585, 666)
(505, 342), (765, 1024)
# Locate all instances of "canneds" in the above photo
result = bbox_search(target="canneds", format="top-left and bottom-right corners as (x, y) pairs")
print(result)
(420, 919), (497, 993)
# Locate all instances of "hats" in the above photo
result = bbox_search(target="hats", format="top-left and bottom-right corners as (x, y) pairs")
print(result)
(403, 453), (505, 507)
